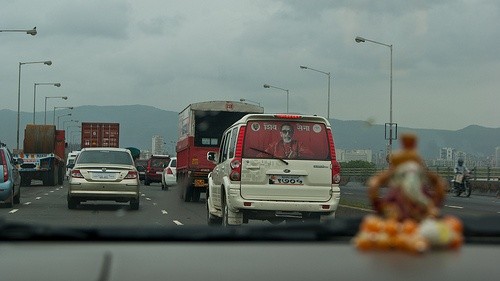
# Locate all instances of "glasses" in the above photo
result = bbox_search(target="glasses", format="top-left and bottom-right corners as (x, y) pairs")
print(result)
(280, 129), (293, 135)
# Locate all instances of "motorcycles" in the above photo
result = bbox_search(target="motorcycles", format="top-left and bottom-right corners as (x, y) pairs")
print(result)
(450, 168), (474, 198)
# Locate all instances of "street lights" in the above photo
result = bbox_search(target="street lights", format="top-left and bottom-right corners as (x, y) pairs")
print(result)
(45, 95), (68, 125)
(57, 113), (80, 143)
(299, 65), (330, 122)
(264, 84), (289, 113)
(33, 81), (61, 124)
(53, 105), (74, 124)
(355, 35), (393, 150)
(16, 60), (53, 149)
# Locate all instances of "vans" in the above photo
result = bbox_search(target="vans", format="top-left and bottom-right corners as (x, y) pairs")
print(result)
(205, 112), (342, 240)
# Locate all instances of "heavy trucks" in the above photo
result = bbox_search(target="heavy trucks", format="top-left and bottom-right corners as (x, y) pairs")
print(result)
(9, 123), (69, 187)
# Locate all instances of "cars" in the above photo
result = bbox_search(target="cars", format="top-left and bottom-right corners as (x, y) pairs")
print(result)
(66, 147), (141, 210)
(66, 150), (81, 169)
(0, 139), (23, 208)
(135, 158), (148, 181)
(161, 157), (177, 190)
(143, 153), (171, 186)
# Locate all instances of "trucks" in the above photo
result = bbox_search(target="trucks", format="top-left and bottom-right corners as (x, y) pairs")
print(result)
(80, 121), (120, 151)
(175, 100), (265, 202)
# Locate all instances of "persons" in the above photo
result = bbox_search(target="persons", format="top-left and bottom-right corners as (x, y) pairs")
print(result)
(453, 158), (471, 189)
(257, 124), (314, 158)
